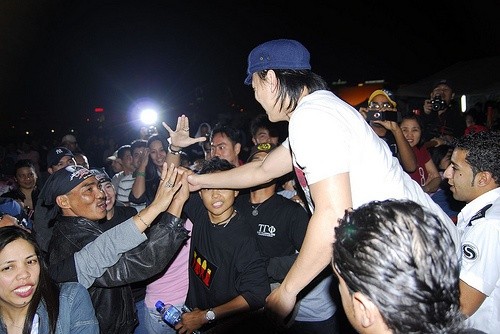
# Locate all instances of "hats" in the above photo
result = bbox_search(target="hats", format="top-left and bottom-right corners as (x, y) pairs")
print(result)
(431, 78), (452, 89)
(107, 150), (119, 160)
(61, 134), (77, 146)
(46, 147), (74, 167)
(246, 143), (277, 163)
(368, 88), (397, 109)
(243, 39), (311, 85)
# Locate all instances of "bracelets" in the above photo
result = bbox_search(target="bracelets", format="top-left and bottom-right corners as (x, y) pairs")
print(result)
(168, 144), (184, 156)
(137, 172), (145, 176)
(136, 214), (151, 228)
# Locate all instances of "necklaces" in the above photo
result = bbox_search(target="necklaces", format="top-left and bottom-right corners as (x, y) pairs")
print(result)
(250, 202), (261, 217)
(206, 213), (235, 230)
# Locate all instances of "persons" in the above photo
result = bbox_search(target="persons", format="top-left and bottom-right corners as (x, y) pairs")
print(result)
(0, 226), (100, 334)
(0, 115), (312, 295)
(162, 114), (271, 334)
(35, 165), (195, 334)
(176, 39), (456, 316)
(331, 200), (491, 334)
(356, 78), (500, 226)
(442, 132), (500, 334)
(132, 217), (194, 334)
(161, 115), (270, 334)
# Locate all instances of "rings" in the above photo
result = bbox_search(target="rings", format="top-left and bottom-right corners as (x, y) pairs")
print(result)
(161, 178), (164, 182)
(183, 126), (191, 132)
(164, 181), (173, 189)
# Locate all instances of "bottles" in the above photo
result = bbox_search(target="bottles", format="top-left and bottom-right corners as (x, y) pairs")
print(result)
(155, 300), (200, 334)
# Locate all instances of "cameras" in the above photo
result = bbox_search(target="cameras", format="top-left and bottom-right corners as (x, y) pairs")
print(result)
(364, 110), (398, 121)
(430, 95), (446, 111)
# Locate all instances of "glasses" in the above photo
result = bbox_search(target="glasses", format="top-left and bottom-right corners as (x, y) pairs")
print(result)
(368, 101), (392, 109)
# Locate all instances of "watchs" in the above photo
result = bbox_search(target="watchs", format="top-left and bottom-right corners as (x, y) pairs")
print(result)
(204, 307), (217, 322)
